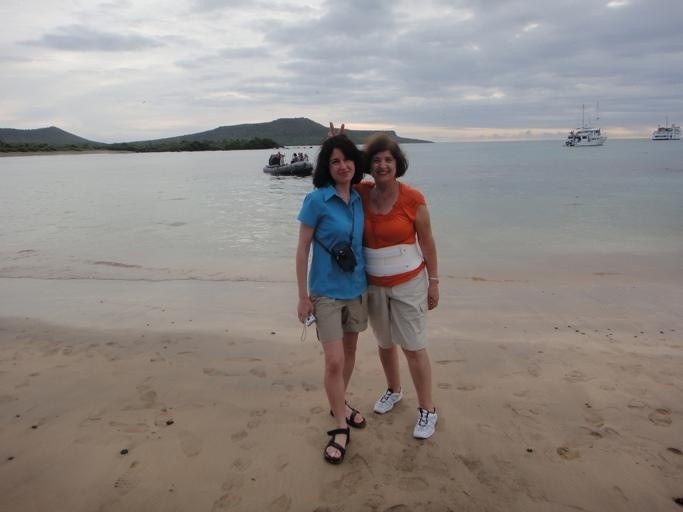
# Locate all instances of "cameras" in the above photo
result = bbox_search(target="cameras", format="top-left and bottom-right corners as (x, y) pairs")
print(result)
(303, 313), (316, 327)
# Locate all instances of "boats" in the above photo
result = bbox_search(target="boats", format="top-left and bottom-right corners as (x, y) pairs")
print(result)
(263, 152), (315, 178)
(650, 114), (682, 140)
(564, 101), (609, 146)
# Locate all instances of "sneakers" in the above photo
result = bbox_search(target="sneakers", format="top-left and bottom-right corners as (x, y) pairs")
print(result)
(373, 385), (402, 414)
(412, 407), (438, 439)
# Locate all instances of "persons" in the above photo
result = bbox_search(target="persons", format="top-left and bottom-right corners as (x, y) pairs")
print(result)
(294, 133), (368, 464)
(326, 119), (441, 440)
(275, 151), (309, 167)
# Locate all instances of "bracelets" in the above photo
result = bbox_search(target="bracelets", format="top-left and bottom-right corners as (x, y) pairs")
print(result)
(427, 277), (439, 284)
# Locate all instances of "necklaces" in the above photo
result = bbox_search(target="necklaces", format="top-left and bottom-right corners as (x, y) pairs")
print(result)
(380, 206), (387, 213)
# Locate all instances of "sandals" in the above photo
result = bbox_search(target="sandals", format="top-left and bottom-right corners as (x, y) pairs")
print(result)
(330, 401), (367, 429)
(324, 427), (350, 466)
